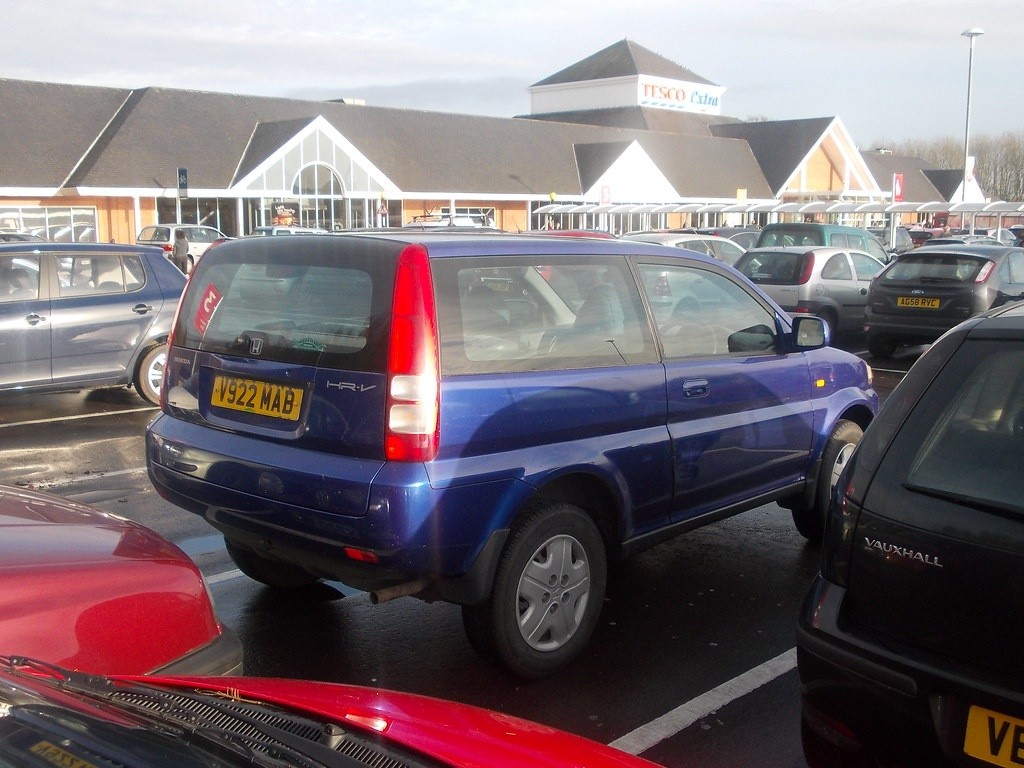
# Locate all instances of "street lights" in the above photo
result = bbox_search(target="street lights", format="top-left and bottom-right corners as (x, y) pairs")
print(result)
(960, 27), (985, 202)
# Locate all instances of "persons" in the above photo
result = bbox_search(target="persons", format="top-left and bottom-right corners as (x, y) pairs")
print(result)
(942, 225), (954, 237)
(172, 230), (190, 276)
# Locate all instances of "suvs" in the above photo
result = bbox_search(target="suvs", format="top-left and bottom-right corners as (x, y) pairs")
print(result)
(0, 242), (189, 407)
(137, 223), (239, 275)
(251, 226), (330, 236)
(145, 225), (883, 688)
(793, 302), (1024, 768)
(404, 213), (496, 229)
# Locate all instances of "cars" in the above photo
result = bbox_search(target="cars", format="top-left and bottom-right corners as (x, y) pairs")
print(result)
(1, 655), (665, 768)
(0, 482), (248, 679)
(461, 223), (1024, 360)
(0, 232), (77, 296)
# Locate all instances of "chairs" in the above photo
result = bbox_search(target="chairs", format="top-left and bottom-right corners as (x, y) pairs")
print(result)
(574, 284), (624, 334)
(751, 264), (776, 284)
(59, 280), (144, 292)
(457, 285), (509, 338)
